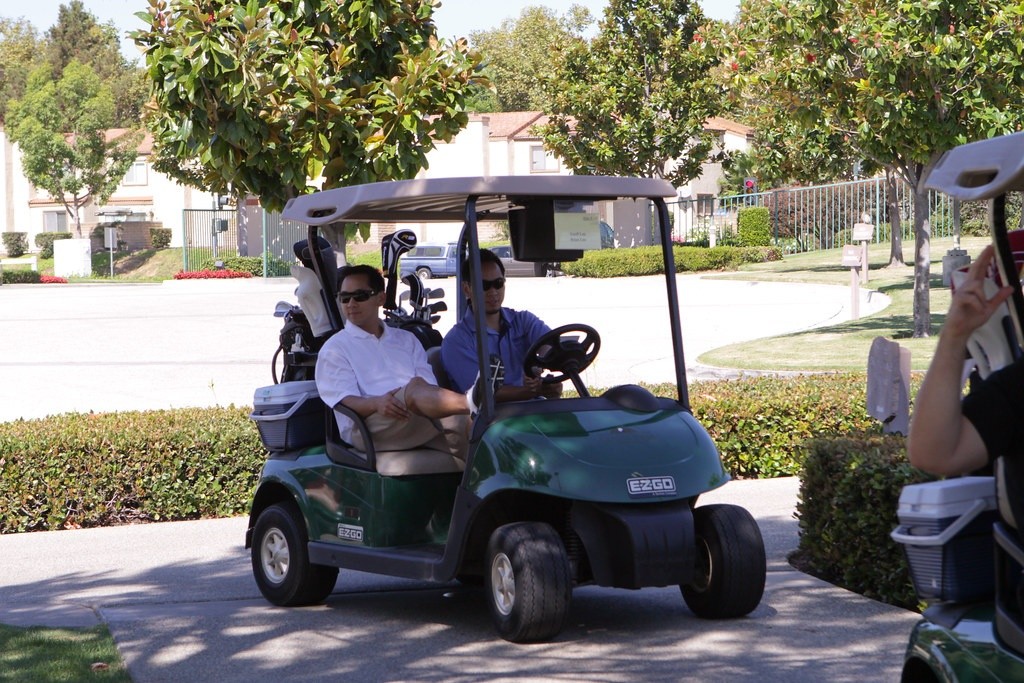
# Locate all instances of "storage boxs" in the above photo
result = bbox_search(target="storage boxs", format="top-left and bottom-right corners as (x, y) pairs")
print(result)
(891, 478), (997, 605)
(251, 380), (325, 452)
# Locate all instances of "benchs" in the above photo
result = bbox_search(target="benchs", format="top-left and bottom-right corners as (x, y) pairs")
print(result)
(322, 400), (465, 477)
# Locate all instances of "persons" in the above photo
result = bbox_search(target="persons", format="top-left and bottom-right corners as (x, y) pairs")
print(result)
(440, 250), (562, 403)
(315, 265), (506, 460)
(907, 246), (1024, 622)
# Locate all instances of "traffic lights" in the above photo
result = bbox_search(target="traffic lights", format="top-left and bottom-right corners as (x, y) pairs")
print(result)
(743, 177), (756, 205)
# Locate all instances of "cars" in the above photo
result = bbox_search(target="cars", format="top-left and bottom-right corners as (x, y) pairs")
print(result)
(398, 241), (547, 280)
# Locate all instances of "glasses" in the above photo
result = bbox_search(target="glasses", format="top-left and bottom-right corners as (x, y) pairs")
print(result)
(482, 277), (506, 291)
(338, 288), (384, 304)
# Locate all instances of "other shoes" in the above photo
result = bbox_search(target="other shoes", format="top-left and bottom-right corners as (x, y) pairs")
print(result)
(465, 369), (495, 420)
